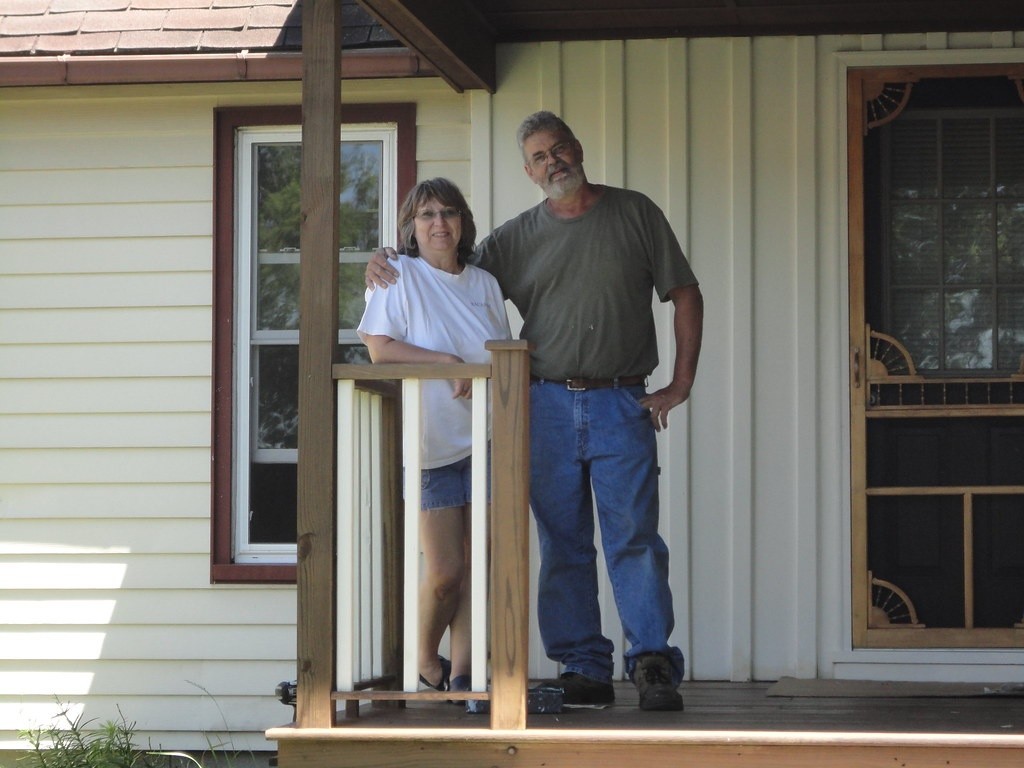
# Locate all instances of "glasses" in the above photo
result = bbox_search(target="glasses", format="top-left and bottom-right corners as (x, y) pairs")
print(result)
(416, 207), (463, 218)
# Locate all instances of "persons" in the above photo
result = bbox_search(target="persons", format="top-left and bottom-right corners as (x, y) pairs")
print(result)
(364, 111), (704, 711)
(355, 176), (513, 706)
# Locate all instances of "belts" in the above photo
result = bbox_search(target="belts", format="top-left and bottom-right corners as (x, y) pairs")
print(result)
(543, 377), (642, 391)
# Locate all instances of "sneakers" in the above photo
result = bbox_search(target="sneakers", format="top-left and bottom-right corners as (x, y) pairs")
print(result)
(536, 672), (614, 709)
(633, 655), (684, 710)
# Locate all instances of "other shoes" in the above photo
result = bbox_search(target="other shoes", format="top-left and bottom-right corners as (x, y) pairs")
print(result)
(449, 675), (472, 704)
(420, 654), (452, 691)
